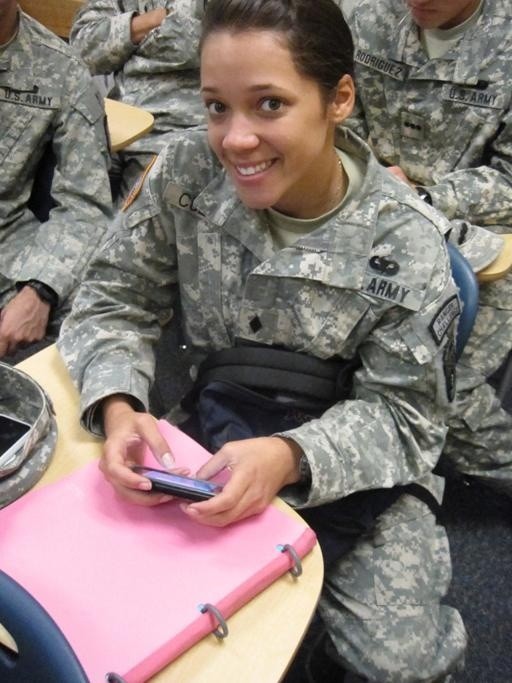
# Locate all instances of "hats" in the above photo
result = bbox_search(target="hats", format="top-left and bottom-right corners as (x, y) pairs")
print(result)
(0, 362), (59, 508)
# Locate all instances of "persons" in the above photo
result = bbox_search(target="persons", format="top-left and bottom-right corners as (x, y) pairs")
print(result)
(68, 0), (206, 209)
(53, 1), (470, 683)
(334, 0), (512, 498)
(0, 0), (111, 363)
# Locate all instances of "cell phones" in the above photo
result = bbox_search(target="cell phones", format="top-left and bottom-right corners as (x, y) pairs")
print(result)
(128, 465), (225, 502)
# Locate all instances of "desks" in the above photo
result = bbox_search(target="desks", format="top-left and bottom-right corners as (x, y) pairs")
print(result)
(0, 340), (325, 683)
(472, 230), (512, 284)
(103, 96), (155, 154)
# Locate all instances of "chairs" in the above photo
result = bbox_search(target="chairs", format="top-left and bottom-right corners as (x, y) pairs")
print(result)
(447, 240), (480, 374)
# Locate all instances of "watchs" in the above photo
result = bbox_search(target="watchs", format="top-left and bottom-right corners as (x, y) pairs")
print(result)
(28, 281), (60, 302)
(417, 188), (433, 208)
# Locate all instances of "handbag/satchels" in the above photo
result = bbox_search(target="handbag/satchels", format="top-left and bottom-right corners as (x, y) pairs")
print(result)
(178, 341), (407, 563)
